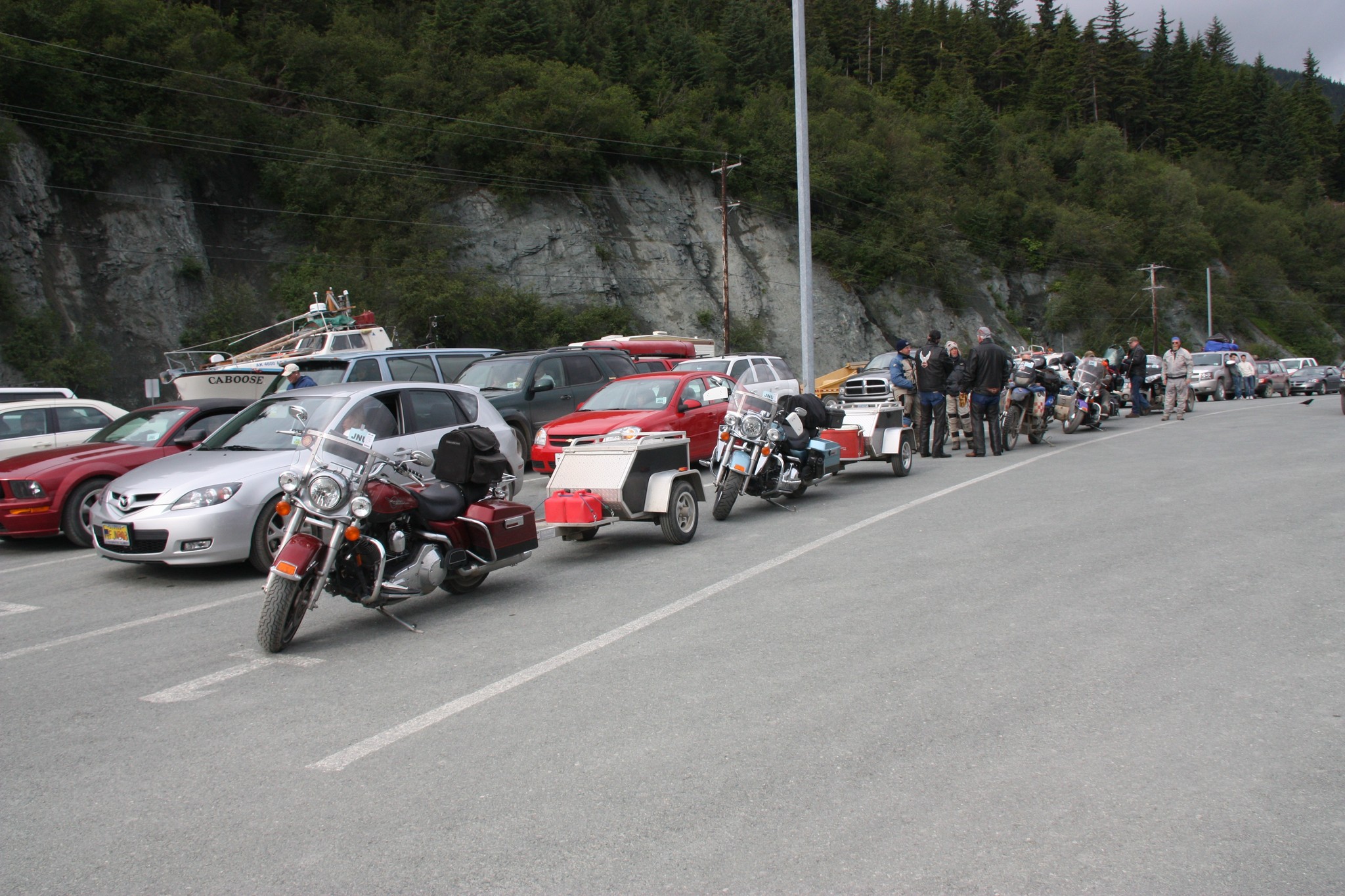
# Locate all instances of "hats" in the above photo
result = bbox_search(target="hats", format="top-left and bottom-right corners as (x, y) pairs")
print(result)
(976, 327), (991, 336)
(281, 363), (299, 376)
(1171, 337), (1179, 342)
(945, 341), (962, 357)
(1085, 351), (1095, 357)
(929, 330), (941, 339)
(1126, 336), (1139, 343)
(896, 340), (910, 351)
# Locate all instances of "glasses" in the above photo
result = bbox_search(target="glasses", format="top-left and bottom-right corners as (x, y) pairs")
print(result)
(905, 346), (912, 349)
(1172, 342), (1179, 344)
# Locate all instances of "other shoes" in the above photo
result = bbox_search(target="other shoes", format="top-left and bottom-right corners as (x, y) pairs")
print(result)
(932, 453), (951, 458)
(912, 449), (919, 454)
(952, 441), (960, 450)
(965, 452), (985, 457)
(967, 440), (975, 449)
(994, 452), (1002, 456)
(1161, 415), (1184, 420)
(1125, 413), (1140, 418)
(1233, 395), (1254, 400)
(1100, 404), (1108, 421)
(1144, 405), (1153, 416)
(920, 452), (932, 457)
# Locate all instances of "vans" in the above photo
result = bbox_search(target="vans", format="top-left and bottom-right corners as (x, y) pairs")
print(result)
(0, 386), (95, 436)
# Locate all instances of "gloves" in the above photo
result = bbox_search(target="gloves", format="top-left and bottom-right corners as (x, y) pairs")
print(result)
(959, 391), (968, 407)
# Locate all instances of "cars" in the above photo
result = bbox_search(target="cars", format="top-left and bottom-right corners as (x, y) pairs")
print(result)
(529, 370), (756, 484)
(1288, 364), (1342, 396)
(82, 378), (526, 579)
(1110, 349), (1164, 411)
(0, 397), (151, 464)
(165, 330), (802, 431)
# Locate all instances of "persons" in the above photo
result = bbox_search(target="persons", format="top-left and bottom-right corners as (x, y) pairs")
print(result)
(1075, 350), (1111, 415)
(280, 363), (318, 391)
(1161, 337), (1194, 421)
(944, 341), (975, 450)
(889, 340), (921, 454)
(915, 329), (955, 458)
(1228, 353), (1245, 400)
(342, 404), (380, 440)
(1121, 336), (1152, 418)
(637, 388), (697, 408)
(205, 415), (222, 436)
(352, 367), (374, 382)
(517, 365), (556, 387)
(1238, 354), (1255, 399)
(959, 326), (1008, 456)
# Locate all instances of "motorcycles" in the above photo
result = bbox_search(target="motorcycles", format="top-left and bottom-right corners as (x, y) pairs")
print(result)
(252, 406), (543, 652)
(999, 346), (1056, 452)
(699, 362), (848, 522)
(1053, 352), (1118, 434)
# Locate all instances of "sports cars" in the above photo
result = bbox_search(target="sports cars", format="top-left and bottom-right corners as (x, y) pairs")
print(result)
(0, 397), (261, 549)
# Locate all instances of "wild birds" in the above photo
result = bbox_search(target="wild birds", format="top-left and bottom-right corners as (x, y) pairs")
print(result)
(1300, 399), (1314, 406)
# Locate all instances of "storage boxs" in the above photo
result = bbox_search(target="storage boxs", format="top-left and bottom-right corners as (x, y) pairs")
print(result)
(820, 424), (864, 458)
(1054, 390), (1077, 421)
(807, 438), (841, 468)
(824, 407), (846, 428)
(463, 499), (538, 563)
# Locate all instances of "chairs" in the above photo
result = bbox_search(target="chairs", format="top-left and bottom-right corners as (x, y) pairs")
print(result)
(367, 408), (388, 439)
(429, 403), (453, 428)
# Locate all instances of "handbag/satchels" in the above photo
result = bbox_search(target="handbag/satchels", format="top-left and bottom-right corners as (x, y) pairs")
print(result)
(778, 393), (826, 429)
(431, 425), (506, 484)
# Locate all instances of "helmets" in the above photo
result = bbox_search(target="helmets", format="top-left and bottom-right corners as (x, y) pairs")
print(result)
(1061, 352), (1076, 363)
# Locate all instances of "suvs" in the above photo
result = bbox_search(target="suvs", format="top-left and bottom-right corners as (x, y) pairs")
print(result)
(839, 343), (925, 405)
(1278, 357), (1319, 377)
(1187, 349), (1258, 402)
(1254, 357), (1290, 398)
(449, 344), (644, 471)
